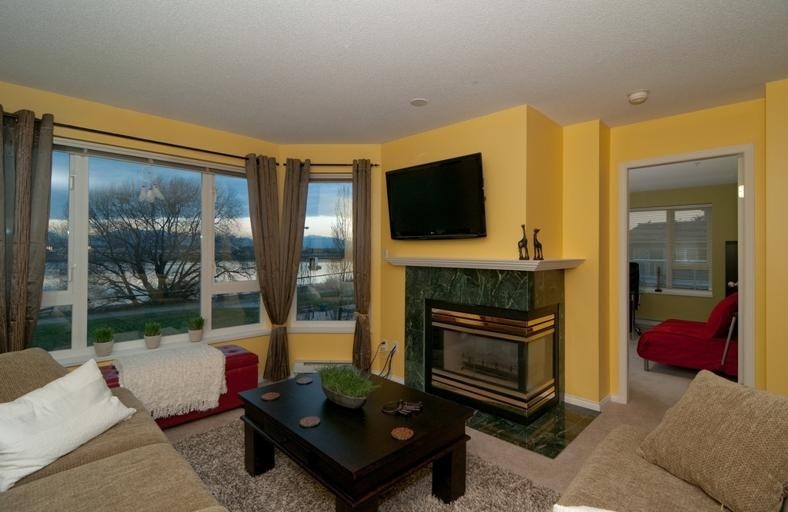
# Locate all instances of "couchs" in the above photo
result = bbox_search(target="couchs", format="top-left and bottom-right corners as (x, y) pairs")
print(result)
(0, 347), (229, 511)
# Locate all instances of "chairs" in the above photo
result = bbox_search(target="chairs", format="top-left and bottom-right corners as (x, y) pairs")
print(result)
(635, 291), (738, 377)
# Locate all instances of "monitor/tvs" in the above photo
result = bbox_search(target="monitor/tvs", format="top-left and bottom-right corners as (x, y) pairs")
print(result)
(385, 152), (487, 241)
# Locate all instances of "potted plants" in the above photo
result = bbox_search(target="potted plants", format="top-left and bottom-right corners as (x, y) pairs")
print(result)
(187, 314), (205, 343)
(143, 321), (163, 350)
(314, 358), (382, 409)
(93, 324), (116, 358)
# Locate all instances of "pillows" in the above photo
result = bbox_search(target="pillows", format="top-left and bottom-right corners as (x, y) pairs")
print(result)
(634, 367), (788, 512)
(0, 358), (137, 492)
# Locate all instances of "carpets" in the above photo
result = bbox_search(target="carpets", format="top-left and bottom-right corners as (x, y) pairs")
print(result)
(170, 418), (563, 512)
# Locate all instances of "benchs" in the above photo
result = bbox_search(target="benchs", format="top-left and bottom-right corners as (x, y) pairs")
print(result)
(99, 344), (260, 430)
